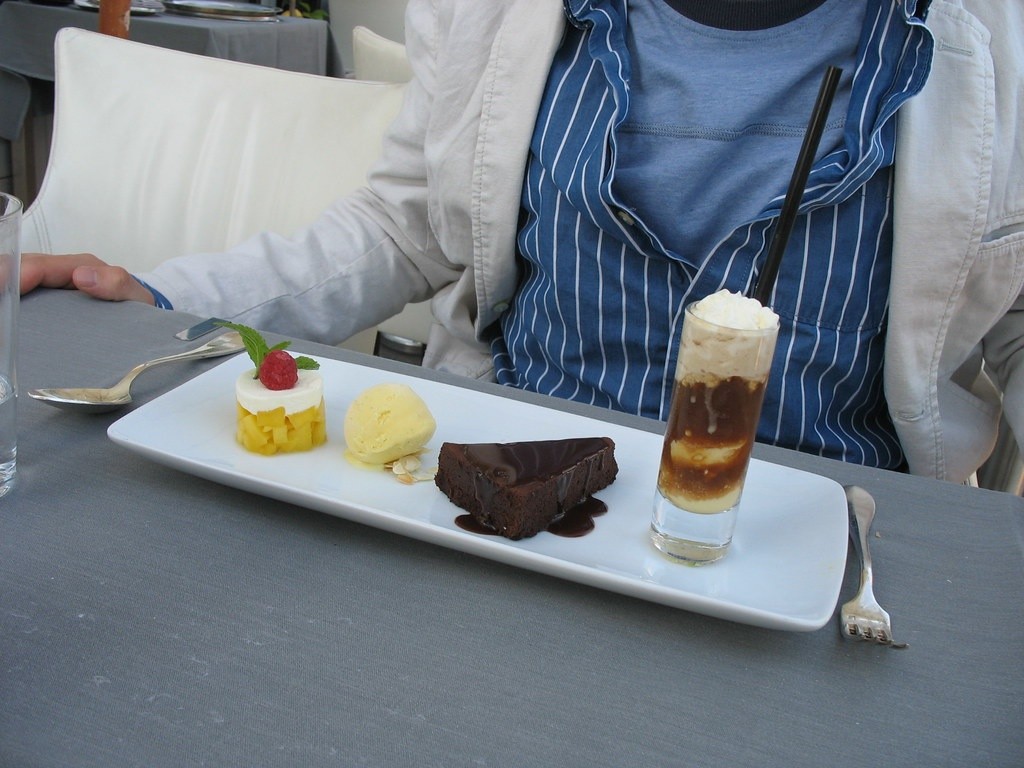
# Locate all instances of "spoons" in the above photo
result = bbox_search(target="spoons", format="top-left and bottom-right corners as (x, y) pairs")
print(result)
(26, 329), (246, 413)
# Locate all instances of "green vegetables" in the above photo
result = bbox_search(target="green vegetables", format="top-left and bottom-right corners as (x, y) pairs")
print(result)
(212, 321), (320, 380)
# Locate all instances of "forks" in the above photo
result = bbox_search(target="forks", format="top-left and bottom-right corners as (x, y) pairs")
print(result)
(840, 484), (910, 649)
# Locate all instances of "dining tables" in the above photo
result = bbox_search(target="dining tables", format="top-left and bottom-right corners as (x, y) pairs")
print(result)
(0, 0), (328, 200)
(0, 289), (1024, 768)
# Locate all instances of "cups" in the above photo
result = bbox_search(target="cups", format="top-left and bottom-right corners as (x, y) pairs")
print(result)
(650, 302), (780, 566)
(0, 191), (23, 500)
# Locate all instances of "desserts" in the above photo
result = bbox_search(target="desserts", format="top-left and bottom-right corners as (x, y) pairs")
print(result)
(343, 380), (436, 465)
(235, 349), (329, 455)
(434, 436), (620, 541)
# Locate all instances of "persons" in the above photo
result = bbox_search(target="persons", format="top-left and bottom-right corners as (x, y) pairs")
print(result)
(1, 0), (1024, 483)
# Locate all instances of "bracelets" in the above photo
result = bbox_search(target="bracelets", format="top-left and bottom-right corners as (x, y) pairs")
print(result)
(130, 273), (174, 310)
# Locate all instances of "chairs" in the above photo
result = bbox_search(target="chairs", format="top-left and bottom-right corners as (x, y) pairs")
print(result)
(0, 27), (437, 365)
(352, 25), (413, 85)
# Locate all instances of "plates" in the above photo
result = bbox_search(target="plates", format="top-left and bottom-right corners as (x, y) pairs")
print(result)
(162, 0), (283, 22)
(105, 350), (849, 632)
(74, 0), (165, 16)
